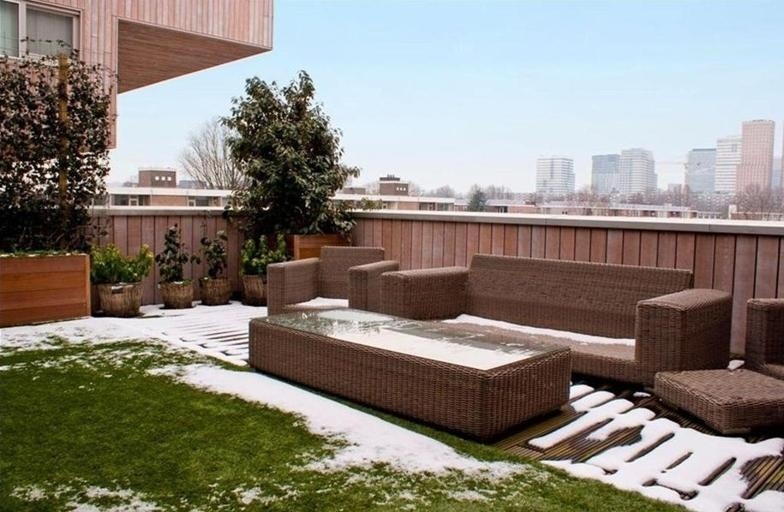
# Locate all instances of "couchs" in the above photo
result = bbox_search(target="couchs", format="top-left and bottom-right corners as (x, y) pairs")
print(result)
(379, 251), (733, 389)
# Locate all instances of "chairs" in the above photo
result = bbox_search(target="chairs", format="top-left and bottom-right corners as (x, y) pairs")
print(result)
(265, 245), (399, 318)
(744, 298), (784, 380)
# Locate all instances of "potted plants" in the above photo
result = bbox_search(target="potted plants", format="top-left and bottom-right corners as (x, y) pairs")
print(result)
(90, 231), (294, 317)
(217, 71), (361, 260)
